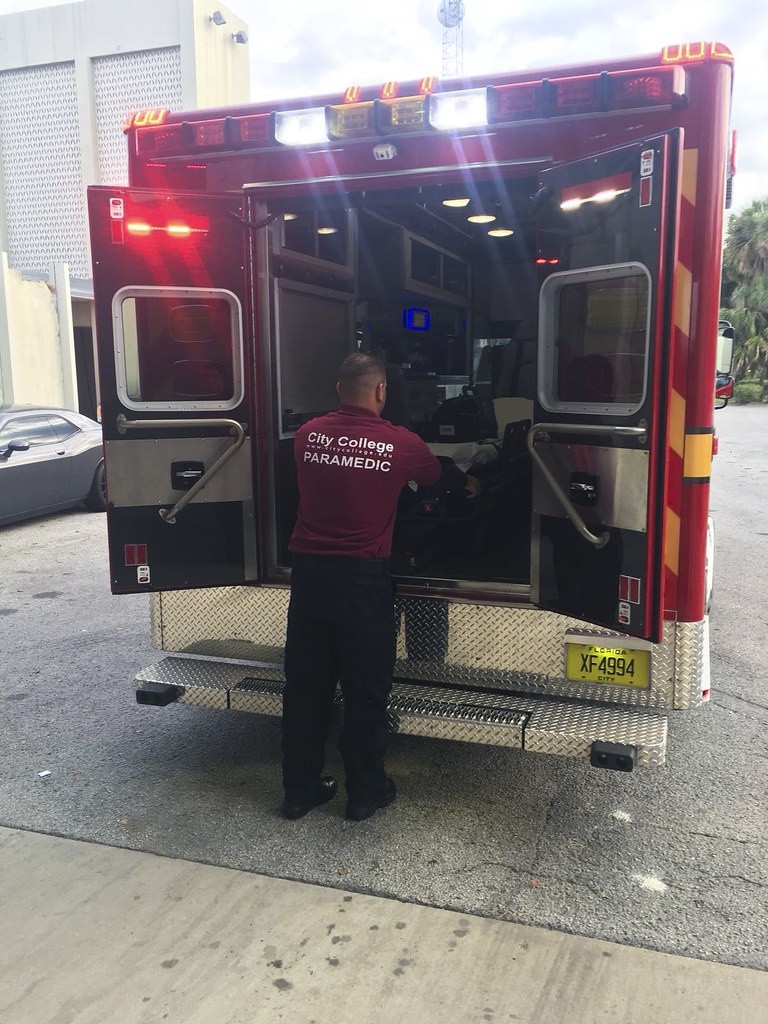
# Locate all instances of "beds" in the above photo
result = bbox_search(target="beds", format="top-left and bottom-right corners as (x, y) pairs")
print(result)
(398, 437), (534, 523)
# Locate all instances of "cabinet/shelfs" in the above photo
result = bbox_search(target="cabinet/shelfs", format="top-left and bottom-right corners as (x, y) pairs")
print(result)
(358, 228), (471, 308)
(273, 202), (358, 274)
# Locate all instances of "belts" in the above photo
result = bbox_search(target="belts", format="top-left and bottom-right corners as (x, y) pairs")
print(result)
(293, 553), (390, 574)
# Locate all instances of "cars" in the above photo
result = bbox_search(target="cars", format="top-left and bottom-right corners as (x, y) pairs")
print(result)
(0, 407), (105, 527)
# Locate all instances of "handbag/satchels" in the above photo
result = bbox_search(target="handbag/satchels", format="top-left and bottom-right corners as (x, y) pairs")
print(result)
(433, 385), (498, 443)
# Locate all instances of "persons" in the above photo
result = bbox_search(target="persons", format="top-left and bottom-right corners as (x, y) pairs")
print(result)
(280, 353), (441, 821)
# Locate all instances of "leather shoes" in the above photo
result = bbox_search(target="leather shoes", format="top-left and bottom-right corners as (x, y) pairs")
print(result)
(282, 776), (337, 818)
(346, 778), (396, 820)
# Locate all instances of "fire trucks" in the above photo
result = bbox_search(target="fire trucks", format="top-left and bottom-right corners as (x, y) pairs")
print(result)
(85, 40), (738, 777)
